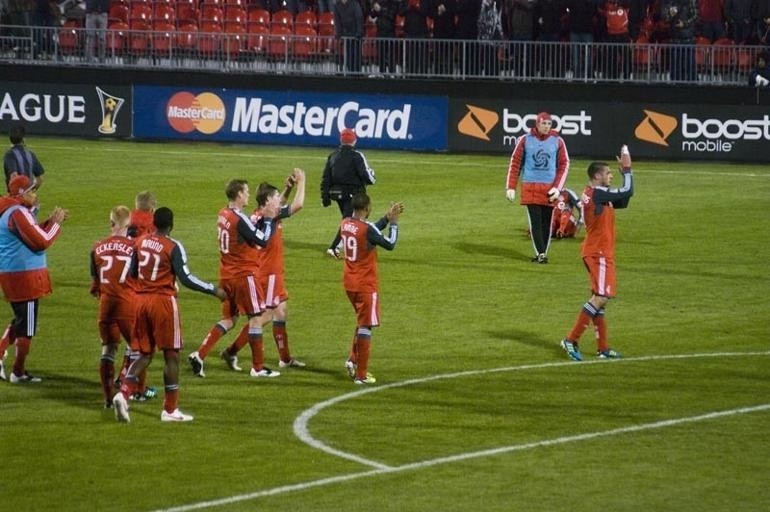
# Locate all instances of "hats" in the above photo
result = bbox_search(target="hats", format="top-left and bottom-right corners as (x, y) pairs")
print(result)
(536, 112), (551, 123)
(9, 172), (36, 198)
(340, 129), (356, 143)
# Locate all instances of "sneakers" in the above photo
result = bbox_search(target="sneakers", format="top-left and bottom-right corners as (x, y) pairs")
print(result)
(345, 360), (355, 378)
(113, 392), (130, 423)
(560, 338), (583, 360)
(189, 351), (205, 377)
(538, 253), (548, 263)
(354, 376), (376, 385)
(327, 248), (340, 259)
(114, 377), (122, 388)
(134, 387), (157, 402)
(161, 408), (193, 422)
(596, 349), (621, 359)
(0, 359), (6, 380)
(251, 367), (281, 377)
(279, 359), (306, 368)
(103, 399), (113, 409)
(10, 372), (42, 384)
(223, 348), (242, 371)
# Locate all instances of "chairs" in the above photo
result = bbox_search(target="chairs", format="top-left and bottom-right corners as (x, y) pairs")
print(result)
(634, 29), (756, 74)
(105, 1), (338, 64)
(361, 13), (436, 61)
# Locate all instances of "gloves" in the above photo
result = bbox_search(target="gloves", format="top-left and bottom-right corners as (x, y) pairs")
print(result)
(506, 189), (515, 202)
(548, 186), (560, 202)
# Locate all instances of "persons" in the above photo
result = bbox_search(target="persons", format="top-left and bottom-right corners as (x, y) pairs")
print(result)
(0, 175), (69, 386)
(3, 125), (45, 197)
(111, 192), (157, 389)
(188, 178), (282, 379)
(559, 144), (635, 360)
(89, 205), (156, 411)
(505, 112), (570, 263)
(112, 208), (227, 423)
(551, 187), (584, 239)
(330, 193), (400, 383)
(220, 166), (306, 373)
(319, 129), (376, 261)
(0, 0), (110, 65)
